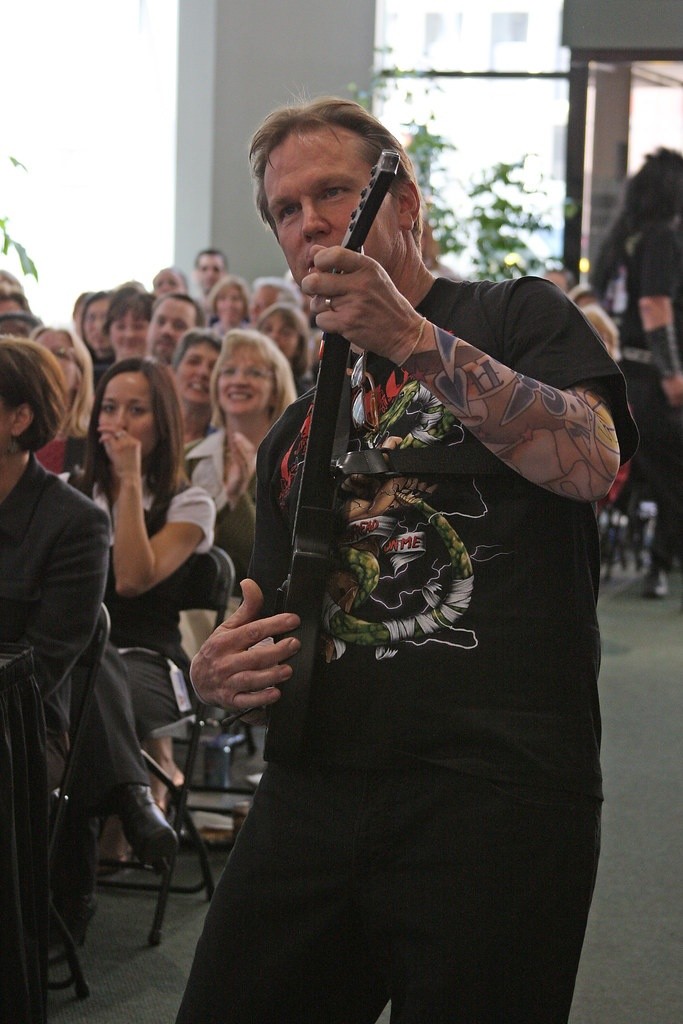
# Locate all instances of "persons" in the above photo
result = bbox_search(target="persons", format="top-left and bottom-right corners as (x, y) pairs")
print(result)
(0, 248), (324, 938)
(178, 99), (641, 1024)
(413, 216), (463, 283)
(544, 148), (683, 603)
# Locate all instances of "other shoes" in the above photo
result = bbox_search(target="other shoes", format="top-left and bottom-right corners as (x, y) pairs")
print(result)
(46, 841), (97, 926)
(205, 721), (248, 751)
(73, 817), (117, 878)
(115, 782), (179, 869)
(641, 566), (667, 597)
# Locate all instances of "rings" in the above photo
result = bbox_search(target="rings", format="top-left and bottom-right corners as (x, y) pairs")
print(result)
(115, 430), (126, 436)
(325, 295), (333, 312)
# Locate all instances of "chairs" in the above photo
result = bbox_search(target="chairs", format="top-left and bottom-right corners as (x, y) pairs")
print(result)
(104, 547), (235, 952)
(45, 603), (110, 1002)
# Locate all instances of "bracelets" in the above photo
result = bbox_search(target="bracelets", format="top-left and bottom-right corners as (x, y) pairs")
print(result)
(397, 316), (427, 371)
(189, 658), (214, 707)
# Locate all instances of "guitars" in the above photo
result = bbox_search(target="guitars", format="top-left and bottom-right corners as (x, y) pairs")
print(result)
(263, 149), (401, 769)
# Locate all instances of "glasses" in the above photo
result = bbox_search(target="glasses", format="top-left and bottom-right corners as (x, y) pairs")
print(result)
(43, 346), (76, 363)
